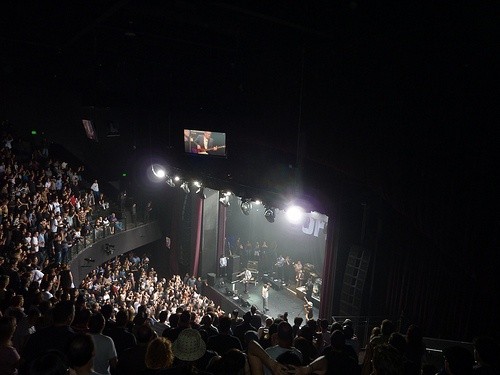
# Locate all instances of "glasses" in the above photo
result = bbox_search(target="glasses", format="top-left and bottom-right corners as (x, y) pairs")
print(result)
(14, 323), (19, 328)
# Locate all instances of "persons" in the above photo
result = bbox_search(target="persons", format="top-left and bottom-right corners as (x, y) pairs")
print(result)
(1, 125), (499, 375)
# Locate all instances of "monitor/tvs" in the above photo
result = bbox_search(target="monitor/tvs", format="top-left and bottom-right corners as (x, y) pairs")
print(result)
(182, 127), (227, 159)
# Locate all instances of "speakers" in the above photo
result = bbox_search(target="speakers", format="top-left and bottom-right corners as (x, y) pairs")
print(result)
(207, 272), (216, 286)
(228, 255), (241, 283)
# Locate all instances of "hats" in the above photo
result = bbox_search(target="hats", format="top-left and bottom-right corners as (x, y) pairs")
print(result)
(342, 319), (354, 325)
(171, 328), (207, 362)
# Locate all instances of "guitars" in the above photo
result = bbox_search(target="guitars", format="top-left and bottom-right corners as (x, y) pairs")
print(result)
(194, 145), (225, 155)
(242, 277), (254, 284)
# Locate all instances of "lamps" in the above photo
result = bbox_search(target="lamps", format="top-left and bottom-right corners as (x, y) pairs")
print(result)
(165, 177), (276, 223)
